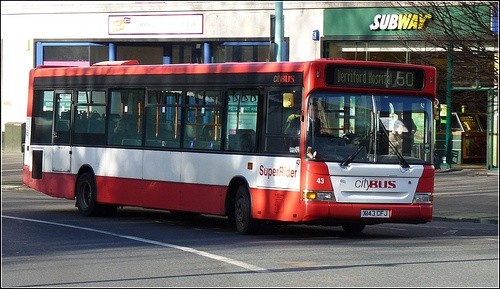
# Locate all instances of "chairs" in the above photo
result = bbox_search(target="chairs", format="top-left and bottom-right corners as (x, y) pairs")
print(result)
(41, 107), (356, 160)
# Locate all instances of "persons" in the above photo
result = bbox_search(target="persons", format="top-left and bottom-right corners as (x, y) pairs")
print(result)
(286, 100), (321, 156)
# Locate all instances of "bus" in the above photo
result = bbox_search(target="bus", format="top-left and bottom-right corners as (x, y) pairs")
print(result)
(24, 59), (441, 236)
